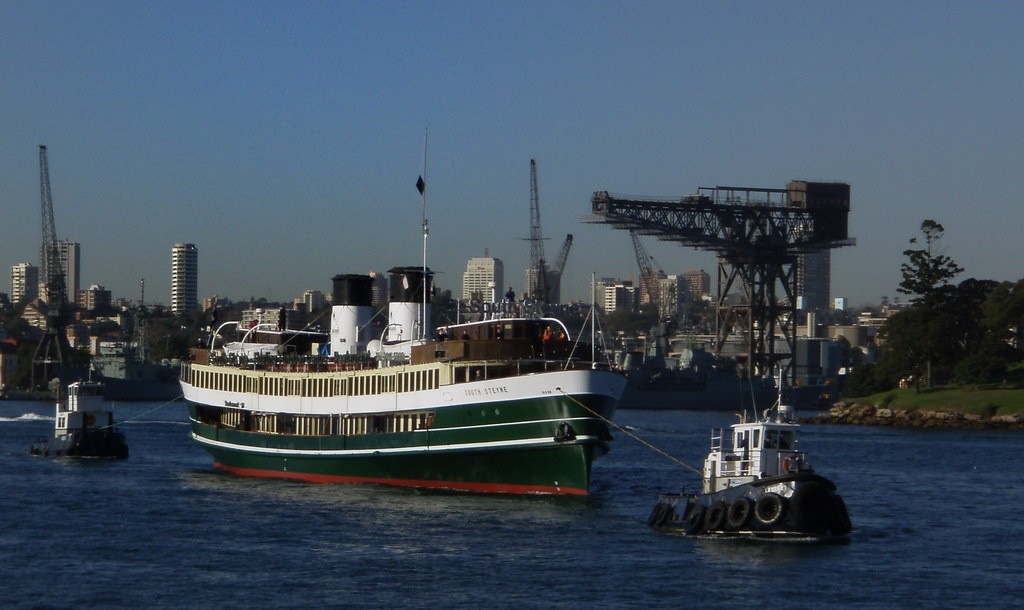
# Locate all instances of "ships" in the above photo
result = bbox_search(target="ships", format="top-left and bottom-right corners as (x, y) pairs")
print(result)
(178, 126), (630, 494)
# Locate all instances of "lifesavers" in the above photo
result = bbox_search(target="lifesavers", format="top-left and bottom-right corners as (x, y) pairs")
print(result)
(784, 456), (803, 474)
(704, 501), (726, 529)
(648, 502), (673, 529)
(755, 492), (783, 524)
(728, 497), (752, 530)
(684, 505), (706, 534)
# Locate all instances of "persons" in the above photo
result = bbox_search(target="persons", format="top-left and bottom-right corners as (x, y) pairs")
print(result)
(764, 431), (789, 449)
(438, 328), (449, 340)
(496, 328), (503, 339)
(557, 327), (567, 341)
(541, 326), (555, 341)
(505, 286), (516, 302)
(462, 329), (470, 340)
(278, 305), (287, 332)
(473, 369), (483, 380)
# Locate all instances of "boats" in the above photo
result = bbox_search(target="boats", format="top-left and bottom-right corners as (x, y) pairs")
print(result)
(27, 376), (132, 461)
(649, 363), (855, 544)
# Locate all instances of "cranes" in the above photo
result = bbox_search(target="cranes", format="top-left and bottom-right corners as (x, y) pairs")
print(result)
(25, 143), (74, 328)
(514, 157), (574, 305)
